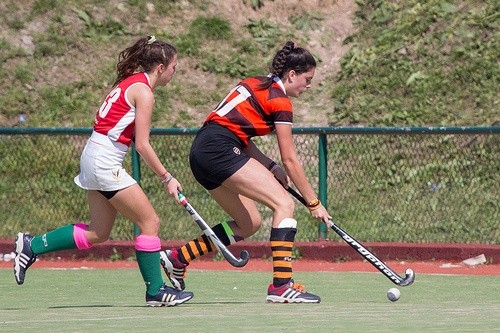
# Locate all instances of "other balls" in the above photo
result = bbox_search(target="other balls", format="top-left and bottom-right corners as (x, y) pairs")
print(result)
(387, 288), (400, 302)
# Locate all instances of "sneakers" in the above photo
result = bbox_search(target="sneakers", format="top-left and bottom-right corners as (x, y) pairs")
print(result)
(159, 247), (188, 291)
(145, 282), (194, 307)
(266, 278), (321, 303)
(14, 232), (37, 285)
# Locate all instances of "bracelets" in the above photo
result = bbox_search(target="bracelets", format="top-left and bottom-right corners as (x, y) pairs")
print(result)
(162, 175), (173, 184)
(159, 171), (168, 177)
(306, 198), (321, 211)
(266, 161), (280, 173)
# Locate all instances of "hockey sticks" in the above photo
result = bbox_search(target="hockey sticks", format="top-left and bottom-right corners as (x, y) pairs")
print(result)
(177, 190), (250, 267)
(276, 178), (415, 287)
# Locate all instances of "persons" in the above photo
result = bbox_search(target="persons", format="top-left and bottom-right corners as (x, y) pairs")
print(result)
(160, 41), (333, 303)
(13, 35), (194, 306)
